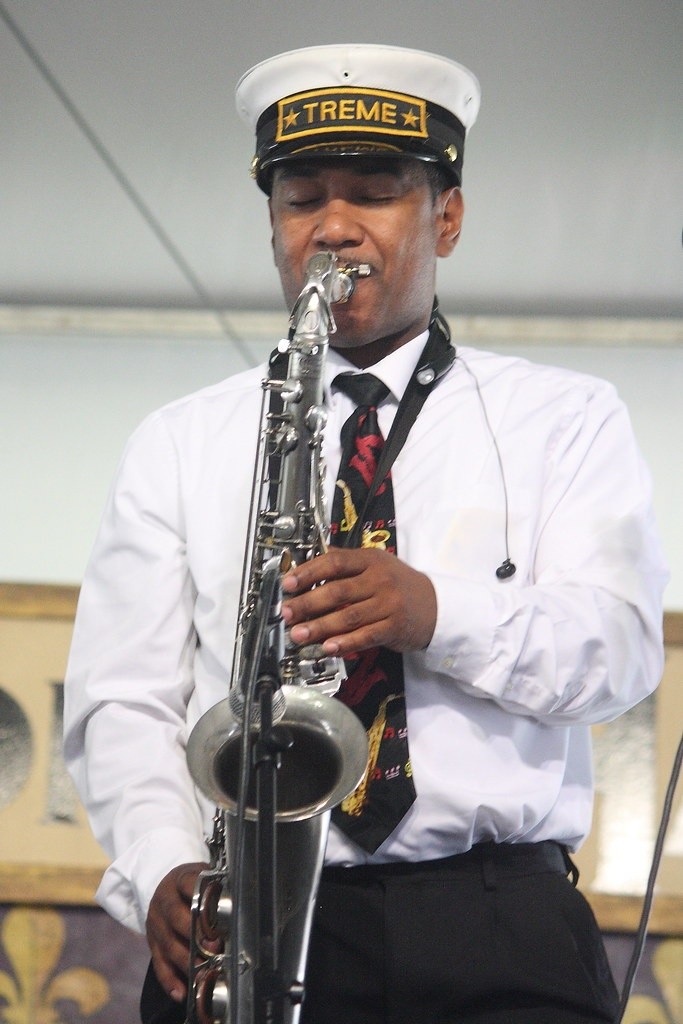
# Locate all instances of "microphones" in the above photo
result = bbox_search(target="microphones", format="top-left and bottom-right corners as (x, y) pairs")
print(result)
(228, 567), (288, 732)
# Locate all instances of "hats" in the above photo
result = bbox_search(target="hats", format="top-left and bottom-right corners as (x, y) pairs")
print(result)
(234, 44), (480, 184)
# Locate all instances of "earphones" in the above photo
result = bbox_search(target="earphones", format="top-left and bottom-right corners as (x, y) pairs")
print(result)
(494, 560), (516, 578)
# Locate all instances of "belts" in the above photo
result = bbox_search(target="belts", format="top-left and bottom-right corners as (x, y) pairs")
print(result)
(313, 842), (570, 878)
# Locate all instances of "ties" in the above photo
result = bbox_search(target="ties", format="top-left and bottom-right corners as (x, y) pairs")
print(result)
(332, 373), (416, 856)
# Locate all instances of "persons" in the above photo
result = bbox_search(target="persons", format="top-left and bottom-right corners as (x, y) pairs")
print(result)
(64, 43), (665, 1024)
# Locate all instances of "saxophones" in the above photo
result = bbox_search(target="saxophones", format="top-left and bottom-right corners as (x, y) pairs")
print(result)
(184, 249), (375, 1023)
